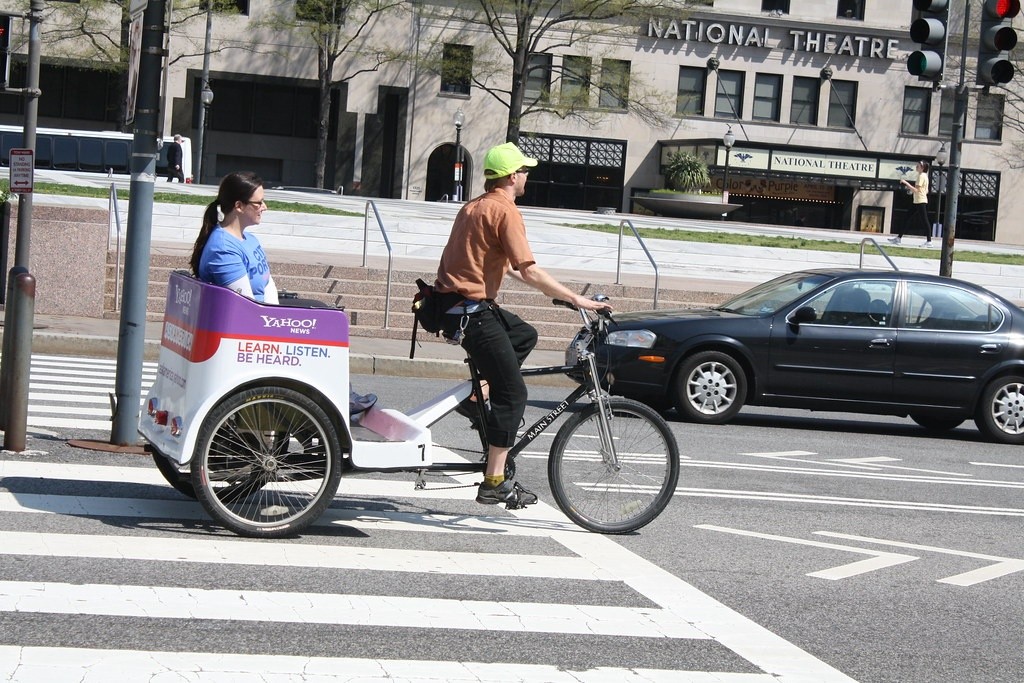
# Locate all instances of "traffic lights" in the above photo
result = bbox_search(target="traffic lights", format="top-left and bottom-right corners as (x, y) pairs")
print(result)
(974, 0), (1021, 87)
(907, 0), (952, 83)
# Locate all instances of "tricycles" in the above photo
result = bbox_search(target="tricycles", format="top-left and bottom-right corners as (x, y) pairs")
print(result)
(137, 269), (681, 539)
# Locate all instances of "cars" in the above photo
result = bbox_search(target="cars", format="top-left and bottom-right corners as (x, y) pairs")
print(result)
(565, 267), (1024, 445)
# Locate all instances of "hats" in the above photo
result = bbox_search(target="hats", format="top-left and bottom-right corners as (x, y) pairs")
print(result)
(483, 142), (538, 179)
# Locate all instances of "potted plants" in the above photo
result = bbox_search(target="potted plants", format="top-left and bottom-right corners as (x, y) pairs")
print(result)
(651, 156), (722, 203)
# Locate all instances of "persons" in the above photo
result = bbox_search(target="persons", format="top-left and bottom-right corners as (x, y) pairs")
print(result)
(433, 141), (614, 506)
(166, 134), (184, 183)
(184, 169), (377, 417)
(887, 160), (934, 248)
(843, 287), (871, 326)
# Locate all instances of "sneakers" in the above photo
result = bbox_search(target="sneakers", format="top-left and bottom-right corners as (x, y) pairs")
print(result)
(457, 398), (489, 422)
(349, 392), (378, 415)
(918, 242), (933, 249)
(888, 237), (902, 246)
(474, 479), (538, 504)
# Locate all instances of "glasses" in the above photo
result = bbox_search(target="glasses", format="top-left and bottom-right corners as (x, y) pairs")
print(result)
(246, 198), (264, 206)
(516, 167), (530, 173)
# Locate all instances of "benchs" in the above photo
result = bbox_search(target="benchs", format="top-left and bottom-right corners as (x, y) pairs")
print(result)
(919, 308), (990, 330)
(168, 270), (350, 378)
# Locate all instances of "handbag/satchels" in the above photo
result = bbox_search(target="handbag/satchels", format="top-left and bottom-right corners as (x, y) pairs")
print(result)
(412, 279), (466, 334)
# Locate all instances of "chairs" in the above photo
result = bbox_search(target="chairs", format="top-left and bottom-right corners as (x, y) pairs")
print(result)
(870, 299), (887, 326)
